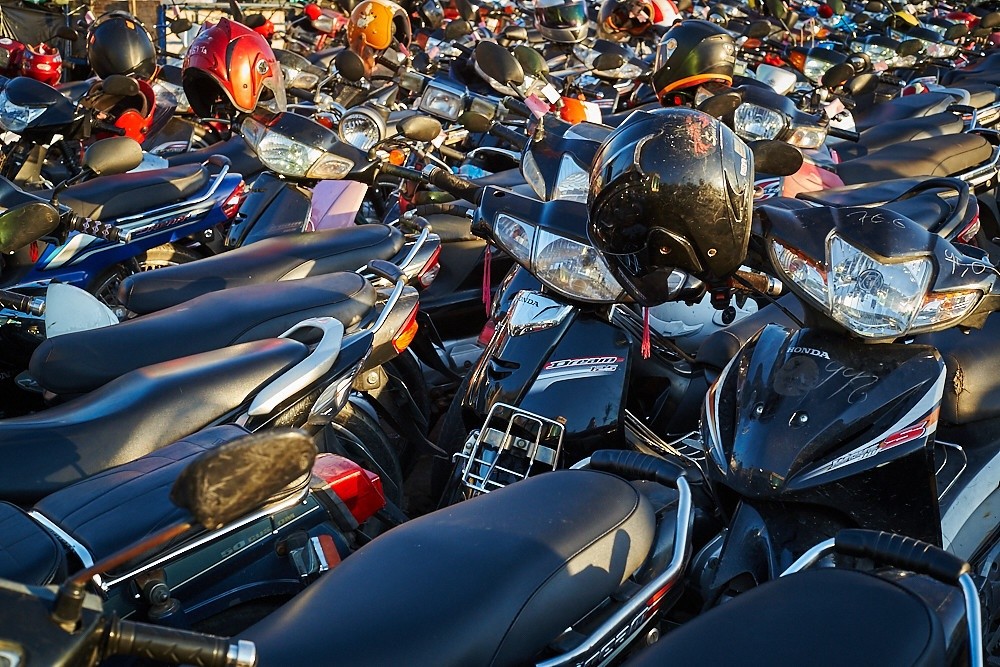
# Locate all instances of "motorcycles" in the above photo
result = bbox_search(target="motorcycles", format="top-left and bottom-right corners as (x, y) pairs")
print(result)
(0, 12), (1000, 667)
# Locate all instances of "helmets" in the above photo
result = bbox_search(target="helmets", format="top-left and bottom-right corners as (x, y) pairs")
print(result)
(347, 1), (412, 65)
(534, 0), (590, 43)
(75, 78), (156, 165)
(181, 17), (279, 118)
(598, 0), (655, 34)
(87, 17), (158, 85)
(585, 107), (755, 309)
(647, 16), (736, 109)
(22, 43), (62, 87)
